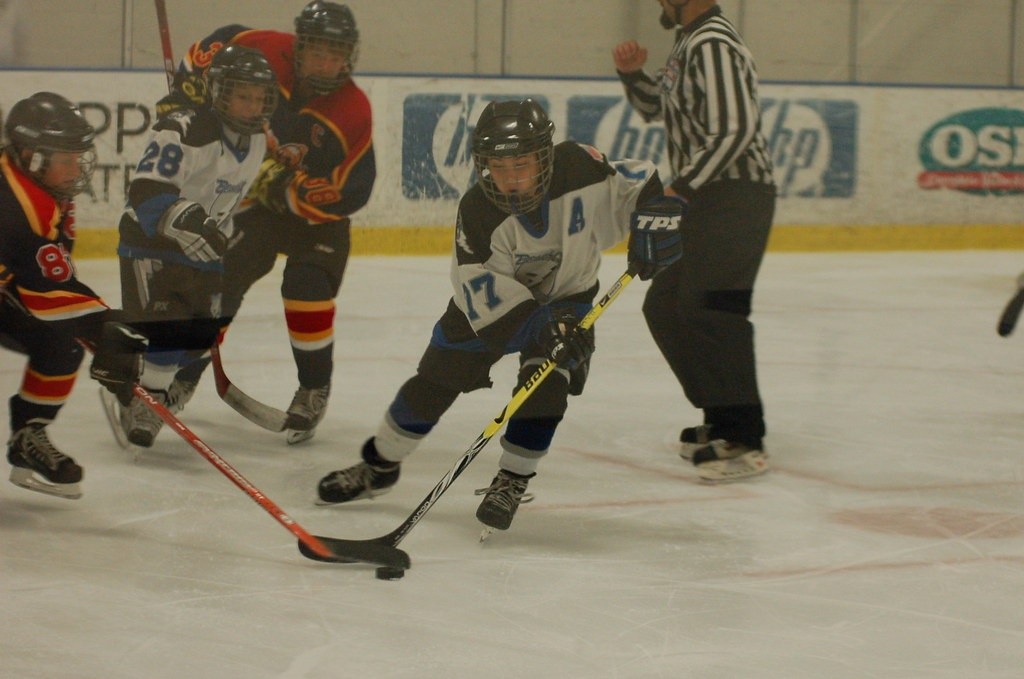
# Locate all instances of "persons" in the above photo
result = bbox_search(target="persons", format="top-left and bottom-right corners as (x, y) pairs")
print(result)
(612, 0), (779, 468)
(318, 98), (683, 530)
(117, 46), (280, 448)
(156, 0), (378, 431)
(0, 92), (153, 483)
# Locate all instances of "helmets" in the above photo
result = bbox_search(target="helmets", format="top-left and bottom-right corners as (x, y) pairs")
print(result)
(5, 92), (98, 198)
(472, 96), (556, 215)
(291, 0), (361, 98)
(207, 45), (279, 136)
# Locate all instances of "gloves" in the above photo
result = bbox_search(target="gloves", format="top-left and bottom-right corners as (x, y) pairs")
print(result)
(157, 196), (228, 264)
(627, 195), (688, 281)
(90, 321), (150, 396)
(155, 75), (213, 120)
(244, 154), (295, 218)
(540, 321), (596, 370)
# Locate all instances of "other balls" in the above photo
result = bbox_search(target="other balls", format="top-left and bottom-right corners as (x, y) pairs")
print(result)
(375, 567), (405, 580)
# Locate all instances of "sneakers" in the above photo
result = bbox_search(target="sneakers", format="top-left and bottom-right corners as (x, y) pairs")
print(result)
(99, 384), (131, 448)
(474, 468), (537, 543)
(120, 359), (172, 447)
(678, 404), (771, 480)
(162, 352), (212, 425)
(285, 367), (331, 443)
(314, 434), (401, 506)
(5, 393), (83, 499)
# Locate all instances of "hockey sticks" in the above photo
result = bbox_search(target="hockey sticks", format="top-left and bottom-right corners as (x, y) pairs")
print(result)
(994, 286), (1023, 338)
(154, 0), (313, 435)
(71, 265), (640, 569)
